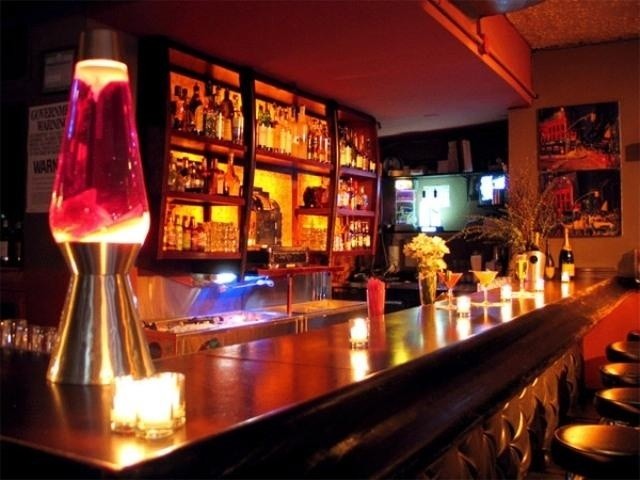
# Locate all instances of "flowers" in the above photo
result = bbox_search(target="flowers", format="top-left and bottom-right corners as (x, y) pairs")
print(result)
(400, 232), (452, 304)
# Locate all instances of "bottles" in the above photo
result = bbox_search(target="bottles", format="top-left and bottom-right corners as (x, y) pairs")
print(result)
(301, 227), (327, 247)
(338, 177), (369, 210)
(162, 212), (241, 252)
(169, 152), (241, 196)
(2, 219), (24, 263)
(334, 220), (371, 250)
(341, 125), (376, 172)
(171, 81), (244, 145)
(559, 228), (575, 282)
(257, 103), (331, 164)
(545, 239), (555, 279)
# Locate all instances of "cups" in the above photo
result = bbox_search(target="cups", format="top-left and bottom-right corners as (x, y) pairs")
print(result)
(366, 277), (386, 322)
(0, 319), (56, 355)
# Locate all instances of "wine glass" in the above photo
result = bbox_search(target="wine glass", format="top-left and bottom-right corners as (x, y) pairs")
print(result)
(515, 255), (529, 295)
(438, 272), (463, 308)
(473, 271), (498, 306)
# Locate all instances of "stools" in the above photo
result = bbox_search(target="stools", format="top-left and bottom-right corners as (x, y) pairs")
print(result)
(549, 325), (640, 479)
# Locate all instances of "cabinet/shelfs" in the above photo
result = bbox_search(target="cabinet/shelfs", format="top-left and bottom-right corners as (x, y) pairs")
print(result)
(136, 35), (382, 265)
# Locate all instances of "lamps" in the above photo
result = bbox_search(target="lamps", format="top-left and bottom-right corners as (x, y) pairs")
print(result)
(40, 21), (160, 391)
